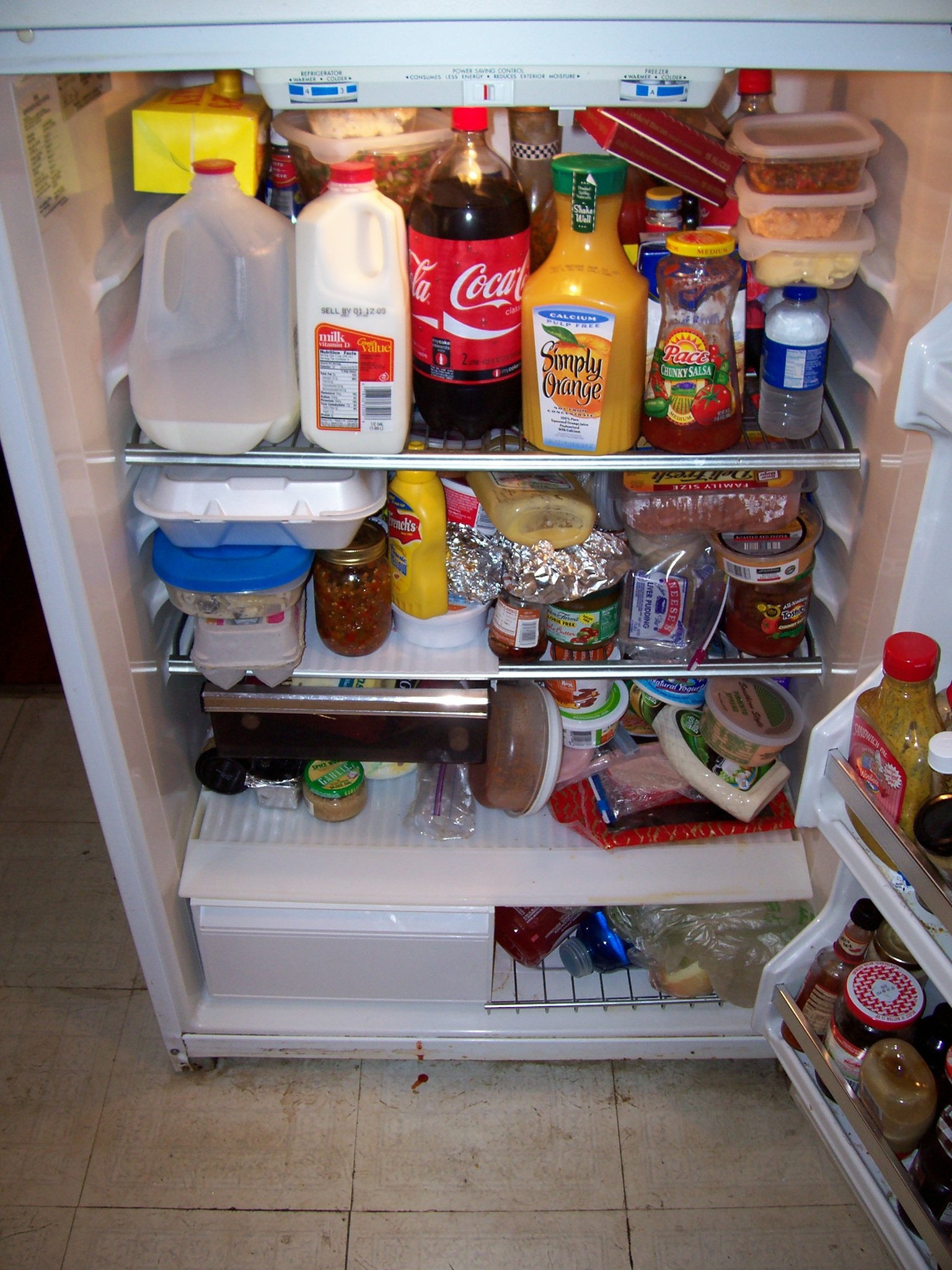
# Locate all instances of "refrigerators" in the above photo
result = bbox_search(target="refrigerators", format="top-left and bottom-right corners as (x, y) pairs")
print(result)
(0, 0), (949, 1270)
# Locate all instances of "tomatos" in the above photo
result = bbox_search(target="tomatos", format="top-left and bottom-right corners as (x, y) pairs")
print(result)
(691, 383), (732, 425)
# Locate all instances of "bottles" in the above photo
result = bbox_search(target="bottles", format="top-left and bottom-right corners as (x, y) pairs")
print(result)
(198, 722), (366, 824)
(847, 631), (951, 841)
(494, 905), (592, 969)
(865, 921), (928, 990)
(386, 469), (815, 660)
(897, 1002), (952, 1244)
(312, 522), (394, 660)
(815, 961), (927, 1103)
(559, 906), (641, 975)
(780, 897), (883, 1053)
(621, 679), (793, 825)
(859, 1036), (936, 1163)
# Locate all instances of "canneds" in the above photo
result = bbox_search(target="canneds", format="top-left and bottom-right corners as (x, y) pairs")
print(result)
(897, 1047), (952, 1239)
(864, 921), (927, 989)
(487, 590), (547, 660)
(724, 553), (816, 656)
(641, 231), (747, 454)
(815, 961), (927, 1102)
(548, 581), (621, 660)
(303, 760), (368, 822)
(313, 521), (393, 656)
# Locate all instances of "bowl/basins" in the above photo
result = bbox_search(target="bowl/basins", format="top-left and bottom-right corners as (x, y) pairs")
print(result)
(275, 108), (453, 208)
(134, 462), (387, 688)
(126, 66), (827, 454)
(703, 499), (824, 582)
(467, 676), (807, 818)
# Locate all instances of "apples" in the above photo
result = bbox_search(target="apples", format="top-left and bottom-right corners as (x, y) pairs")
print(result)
(655, 962), (711, 996)
